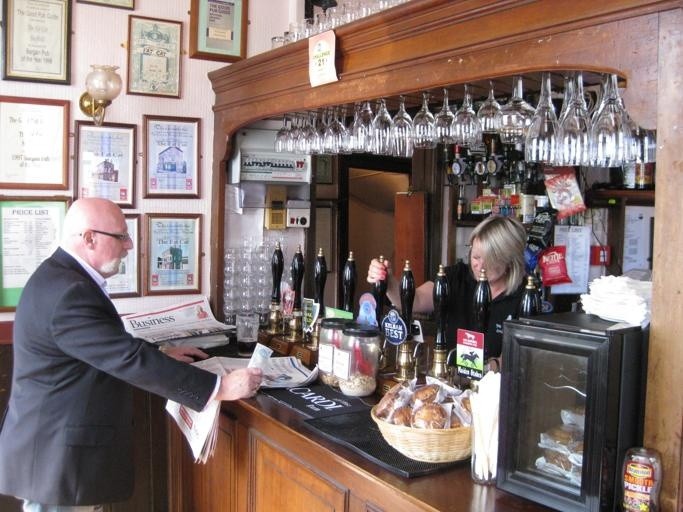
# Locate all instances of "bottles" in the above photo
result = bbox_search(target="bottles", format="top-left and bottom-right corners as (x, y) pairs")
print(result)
(338, 325), (378, 396)
(319, 318), (354, 390)
(472, 153), (489, 184)
(620, 446), (664, 512)
(485, 138), (504, 177)
(446, 145), (470, 184)
(453, 185), (470, 221)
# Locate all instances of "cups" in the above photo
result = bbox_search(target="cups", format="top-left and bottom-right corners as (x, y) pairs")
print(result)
(236, 313), (259, 356)
(223, 236), (294, 322)
(270, 0), (408, 49)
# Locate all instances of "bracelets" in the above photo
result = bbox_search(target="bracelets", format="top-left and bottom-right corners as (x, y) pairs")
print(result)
(159, 345), (167, 353)
(491, 358), (501, 374)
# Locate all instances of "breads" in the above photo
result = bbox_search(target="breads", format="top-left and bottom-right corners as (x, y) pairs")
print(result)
(375, 380), (472, 428)
(541, 401), (590, 472)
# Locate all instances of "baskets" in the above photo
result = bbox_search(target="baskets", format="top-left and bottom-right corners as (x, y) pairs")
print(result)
(370, 403), (472, 465)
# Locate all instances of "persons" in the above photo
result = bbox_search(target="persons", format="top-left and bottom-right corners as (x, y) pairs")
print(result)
(366, 214), (541, 374)
(0, 197), (263, 512)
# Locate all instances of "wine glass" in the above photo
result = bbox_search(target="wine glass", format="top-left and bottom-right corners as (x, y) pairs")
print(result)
(590, 70), (634, 167)
(390, 95), (414, 157)
(434, 89), (455, 143)
(477, 81), (501, 134)
(273, 103), (372, 155)
(413, 93), (438, 150)
(559, 70), (592, 168)
(452, 85), (482, 147)
(525, 71), (562, 162)
(372, 101), (393, 155)
(494, 77), (537, 143)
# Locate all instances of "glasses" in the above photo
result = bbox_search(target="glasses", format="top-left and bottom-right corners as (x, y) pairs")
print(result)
(78, 229), (129, 242)
